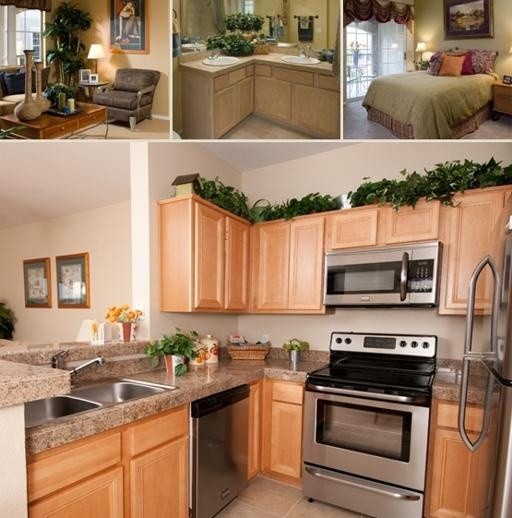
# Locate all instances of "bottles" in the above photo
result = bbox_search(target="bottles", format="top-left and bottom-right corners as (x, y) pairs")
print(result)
(315, 46), (333, 63)
(57, 94), (76, 114)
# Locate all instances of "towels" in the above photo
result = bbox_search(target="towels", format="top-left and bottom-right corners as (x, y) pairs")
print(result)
(270, 13), (283, 37)
(298, 16), (313, 42)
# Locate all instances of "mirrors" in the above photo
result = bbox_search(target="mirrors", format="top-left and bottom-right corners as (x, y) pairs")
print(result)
(179, 0), (283, 58)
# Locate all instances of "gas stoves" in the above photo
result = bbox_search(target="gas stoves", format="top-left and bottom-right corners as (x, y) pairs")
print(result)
(303, 364), (435, 407)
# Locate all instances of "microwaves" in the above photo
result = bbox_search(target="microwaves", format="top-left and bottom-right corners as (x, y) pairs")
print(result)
(322, 239), (443, 309)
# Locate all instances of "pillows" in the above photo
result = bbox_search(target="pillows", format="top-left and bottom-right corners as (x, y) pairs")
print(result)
(18, 66), (50, 92)
(439, 55), (465, 76)
(428, 53), (447, 75)
(5, 73), (31, 94)
(450, 52), (474, 74)
(469, 48), (496, 73)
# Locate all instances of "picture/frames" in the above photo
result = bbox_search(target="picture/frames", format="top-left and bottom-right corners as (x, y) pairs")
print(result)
(443, 0), (493, 38)
(106, 0), (149, 54)
(23, 257), (52, 307)
(55, 253), (90, 308)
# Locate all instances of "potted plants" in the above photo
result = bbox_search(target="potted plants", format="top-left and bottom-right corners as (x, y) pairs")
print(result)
(144, 326), (201, 376)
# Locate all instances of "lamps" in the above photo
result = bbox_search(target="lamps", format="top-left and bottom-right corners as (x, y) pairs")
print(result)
(86, 43), (105, 73)
(415, 42), (428, 69)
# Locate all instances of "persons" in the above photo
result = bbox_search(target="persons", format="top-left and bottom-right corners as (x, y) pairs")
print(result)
(113, 0), (140, 41)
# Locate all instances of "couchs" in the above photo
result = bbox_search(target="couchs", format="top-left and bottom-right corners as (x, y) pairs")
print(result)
(0, 64), (44, 116)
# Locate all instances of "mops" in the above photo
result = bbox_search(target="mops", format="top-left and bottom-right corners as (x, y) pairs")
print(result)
(75, 319), (97, 342)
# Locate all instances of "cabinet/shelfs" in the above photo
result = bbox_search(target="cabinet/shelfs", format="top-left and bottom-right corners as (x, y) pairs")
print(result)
(156, 182), (511, 316)
(26, 378), (501, 518)
(179, 59), (339, 139)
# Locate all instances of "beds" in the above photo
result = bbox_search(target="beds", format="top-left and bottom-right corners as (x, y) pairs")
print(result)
(0, 102), (108, 139)
(362, 69), (501, 139)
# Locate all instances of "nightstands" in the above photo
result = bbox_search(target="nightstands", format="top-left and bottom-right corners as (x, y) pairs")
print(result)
(492, 80), (512, 116)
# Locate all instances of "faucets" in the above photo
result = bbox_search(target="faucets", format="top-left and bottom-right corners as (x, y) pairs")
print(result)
(53, 352), (106, 377)
(301, 46), (311, 58)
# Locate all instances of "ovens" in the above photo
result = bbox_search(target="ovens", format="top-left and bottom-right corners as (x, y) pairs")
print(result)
(301, 390), (429, 491)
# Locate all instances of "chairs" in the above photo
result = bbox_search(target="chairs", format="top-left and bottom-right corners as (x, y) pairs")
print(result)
(93, 68), (161, 129)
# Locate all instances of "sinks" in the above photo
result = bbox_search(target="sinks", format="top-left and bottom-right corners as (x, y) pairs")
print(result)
(67, 377), (180, 402)
(24, 396), (103, 427)
(182, 43), (205, 49)
(202, 56), (240, 66)
(280, 55), (320, 65)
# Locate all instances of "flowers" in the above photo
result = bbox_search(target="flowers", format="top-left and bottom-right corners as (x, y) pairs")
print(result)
(105, 304), (141, 324)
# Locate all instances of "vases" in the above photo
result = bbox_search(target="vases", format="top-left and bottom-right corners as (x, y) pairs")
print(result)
(14, 51), (42, 119)
(119, 322), (132, 342)
(32, 61), (51, 111)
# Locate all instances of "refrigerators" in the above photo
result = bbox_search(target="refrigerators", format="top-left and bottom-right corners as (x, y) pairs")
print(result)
(454, 204), (511, 517)
